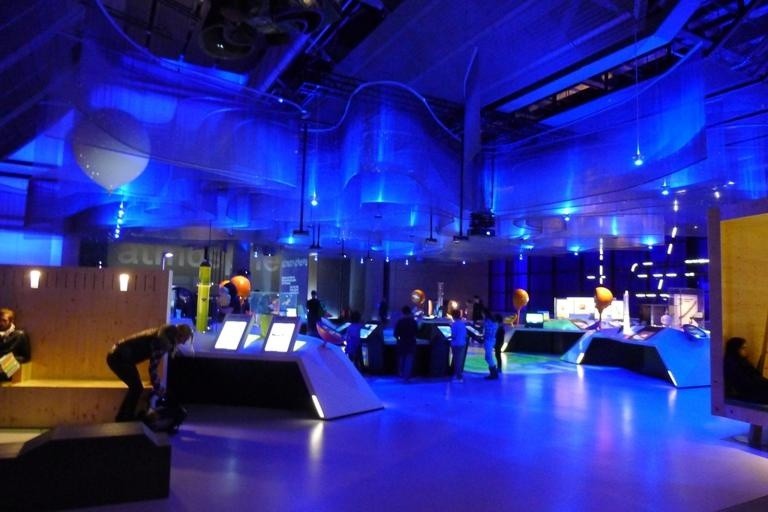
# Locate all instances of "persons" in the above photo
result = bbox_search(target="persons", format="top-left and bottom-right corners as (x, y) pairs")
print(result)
(660, 311), (673, 328)
(378, 294), (504, 382)
(306, 292), (324, 331)
(724, 337), (768, 405)
(106, 324), (194, 421)
(0, 308), (31, 382)
(346, 310), (370, 370)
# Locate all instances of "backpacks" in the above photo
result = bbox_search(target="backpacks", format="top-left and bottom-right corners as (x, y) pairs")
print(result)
(140, 396), (189, 433)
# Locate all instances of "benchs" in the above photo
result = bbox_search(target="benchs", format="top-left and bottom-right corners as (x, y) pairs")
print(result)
(724, 399), (768, 449)
(0, 380), (156, 428)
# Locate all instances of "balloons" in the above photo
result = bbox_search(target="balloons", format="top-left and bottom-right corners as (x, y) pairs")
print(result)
(72, 109), (151, 191)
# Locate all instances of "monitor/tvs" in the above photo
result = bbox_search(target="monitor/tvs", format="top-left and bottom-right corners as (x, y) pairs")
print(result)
(210, 313), (254, 352)
(525, 313), (544, 324)
(337, 322), (379, 338)
(466, 325), (480, 336)
(261, 316), (302, 354)
(436, 326), (452, 337)
(707, 197), (768, 427)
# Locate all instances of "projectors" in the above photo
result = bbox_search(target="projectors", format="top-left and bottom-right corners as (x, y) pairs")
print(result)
(468, 228), (495, 238)
